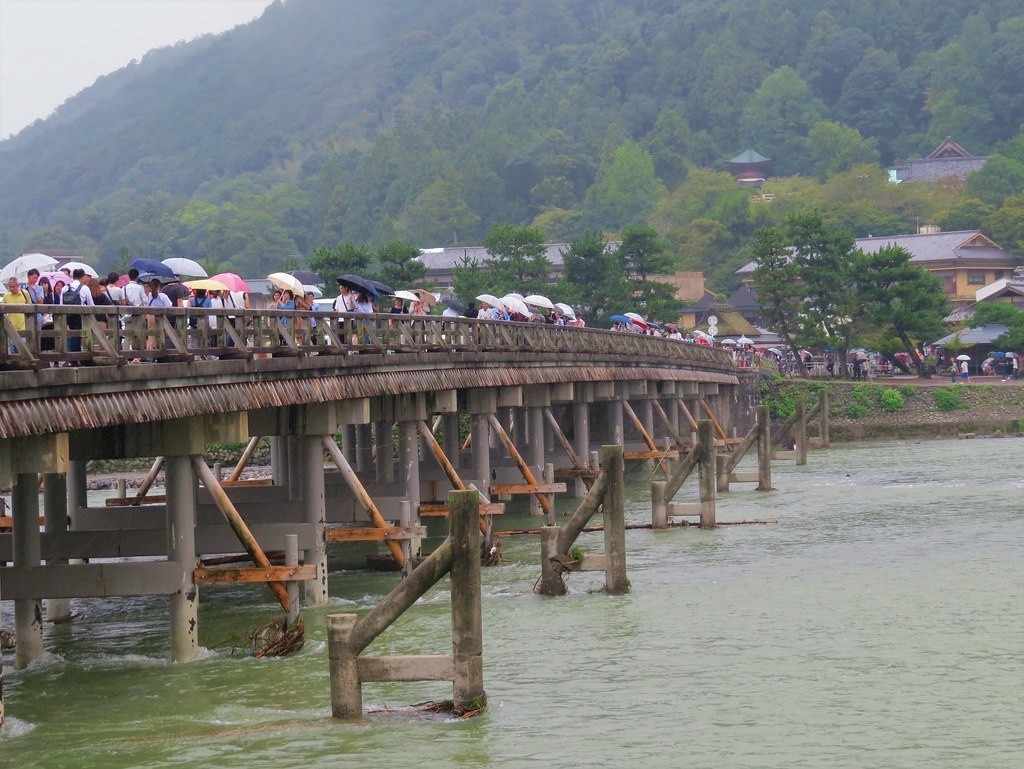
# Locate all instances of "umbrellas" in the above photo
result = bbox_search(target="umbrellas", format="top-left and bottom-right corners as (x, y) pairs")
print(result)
(768, 348), (782, 356)
(442, 293), (575, 318)
(849, 347), (867, 355)
(737, 338), (754, 344)
(692, 330), (709, 340)
(956, 355), (970, 361)
(267, 270), (437, 307)
(610, 312), (677, 333)
(721, 339), (736, 344)
(0, 252), (252, 293)
(992, 352), (1018, 358)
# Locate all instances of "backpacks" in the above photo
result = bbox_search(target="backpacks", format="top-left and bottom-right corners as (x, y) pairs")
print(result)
(62, 283), (85, 329)
(189, 297), (206, 328)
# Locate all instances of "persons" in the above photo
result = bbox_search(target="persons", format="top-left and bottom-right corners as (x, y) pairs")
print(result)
(610, 319), (812, 376)
(464, 301), (585, 332)
(266, 285), (378, 354)
(934, 349), (941, 376)
(826, 352), (834, 378)
(389, 293), (431, 343)
(1, 268), (250, 366)
(443, 305), (459, 344)
(854, 352), (875, 382)
(949, 357), (1019, 383)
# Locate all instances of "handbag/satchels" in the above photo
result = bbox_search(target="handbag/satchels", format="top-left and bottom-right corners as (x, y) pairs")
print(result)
(348, 309), (354, 318)
(42, 313), (53, 327)
(124, 298), (133, 306)
